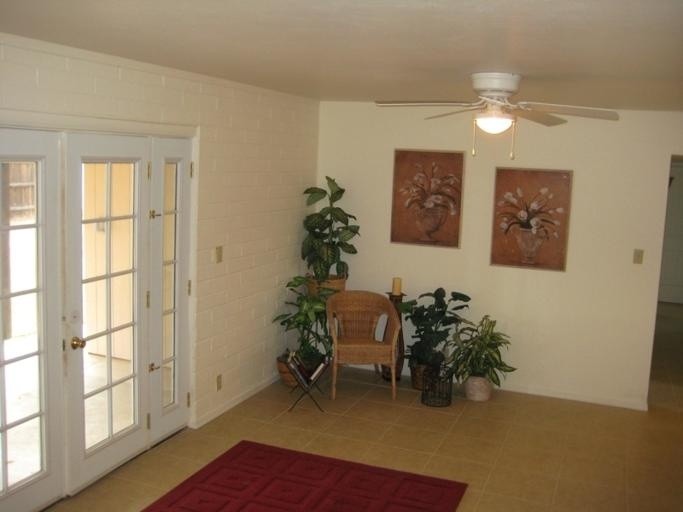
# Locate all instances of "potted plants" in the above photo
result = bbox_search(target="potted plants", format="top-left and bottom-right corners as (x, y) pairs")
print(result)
(396, 287), (472, 390)
(270, 275), (340, 387)
(440, 314), (517, 403)
(296, 175), (361, 303)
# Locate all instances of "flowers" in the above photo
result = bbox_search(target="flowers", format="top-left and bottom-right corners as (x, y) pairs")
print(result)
(496, 187), (564, 241)
(399, 160), (461, 216)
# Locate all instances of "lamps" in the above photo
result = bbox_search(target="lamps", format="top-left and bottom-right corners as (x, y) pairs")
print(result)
(471, 102), (516, 160)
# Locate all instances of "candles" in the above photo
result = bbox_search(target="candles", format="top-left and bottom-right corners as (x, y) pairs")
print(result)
(393, 275), (403, 296)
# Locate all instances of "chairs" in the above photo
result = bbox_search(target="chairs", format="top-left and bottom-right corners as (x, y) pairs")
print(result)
(325, 290), (402, 400)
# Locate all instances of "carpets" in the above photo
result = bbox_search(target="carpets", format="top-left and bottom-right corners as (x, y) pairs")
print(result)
(134, 440), (469, 512)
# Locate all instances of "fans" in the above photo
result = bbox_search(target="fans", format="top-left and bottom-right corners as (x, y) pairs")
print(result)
(373, 73), (619, 160)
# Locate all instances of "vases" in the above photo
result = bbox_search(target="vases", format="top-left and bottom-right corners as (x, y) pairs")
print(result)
(413, 204), (447, 243)
(508, 227), (545, 267)
